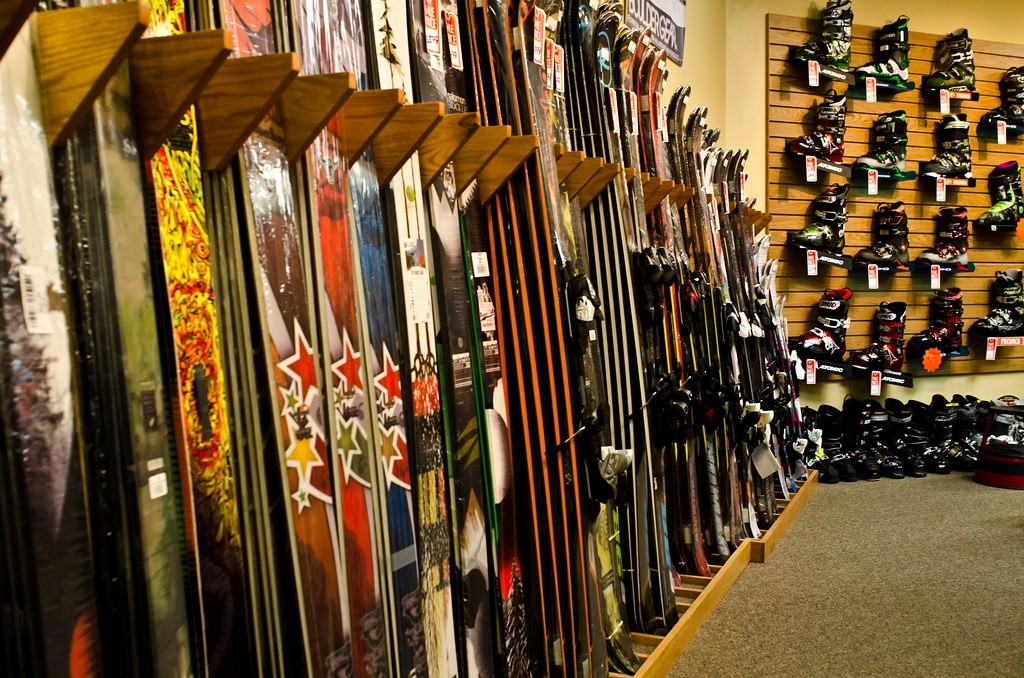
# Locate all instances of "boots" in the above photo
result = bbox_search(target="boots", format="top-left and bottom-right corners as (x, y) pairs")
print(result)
(907, 287), (964, 354)
(852, 110), (908, 173)
(921, 113), (973, 179)
(849, 301), (907, 375)
(784, 89), (848, 165)
(792, 0), (855, 73)
(914, 205), (971, 272)
(852, 199), (912, 271)
(921, 27), (976, 91)
(973, 160), (1024, 228)
(967, 268), (1024, 339)
(802, 393), (1024, 484)
(788, 183), (850, 258)
(980, 65), (1024, 128)
(787, 287), (853, 362)
(852, 14), (911, 88)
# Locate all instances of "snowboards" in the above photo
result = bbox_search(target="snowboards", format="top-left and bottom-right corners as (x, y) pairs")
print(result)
(0, 0), (807, 678)
